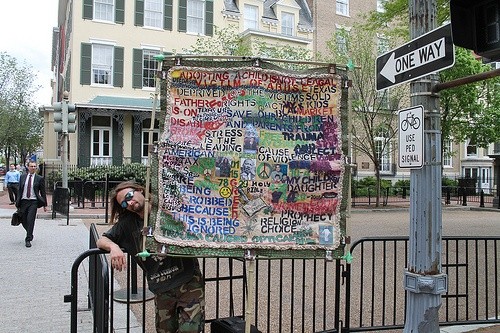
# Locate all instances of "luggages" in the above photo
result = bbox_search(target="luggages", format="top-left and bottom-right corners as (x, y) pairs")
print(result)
(210, 316), (262, 332)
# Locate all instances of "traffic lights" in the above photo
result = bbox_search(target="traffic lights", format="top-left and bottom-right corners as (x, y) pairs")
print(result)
(68, 104), (77, 134)
(54, 101), (63, 133)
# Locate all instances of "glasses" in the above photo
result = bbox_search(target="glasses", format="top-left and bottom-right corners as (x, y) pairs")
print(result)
(121, 189), (136, 210)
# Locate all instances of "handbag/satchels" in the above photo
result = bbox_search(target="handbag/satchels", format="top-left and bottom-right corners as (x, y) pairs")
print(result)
(11, 209), (21, 226)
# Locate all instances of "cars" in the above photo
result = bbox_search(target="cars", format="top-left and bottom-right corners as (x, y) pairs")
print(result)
(0, 166), (7, 176)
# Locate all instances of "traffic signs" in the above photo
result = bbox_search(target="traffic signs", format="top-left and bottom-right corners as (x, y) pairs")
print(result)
(373, 21), (455, 92)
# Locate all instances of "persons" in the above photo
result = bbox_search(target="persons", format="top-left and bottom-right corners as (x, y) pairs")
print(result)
(15, 161), (47, 247)
(96, 181), (205, 333)
(4, 154), (44, 205)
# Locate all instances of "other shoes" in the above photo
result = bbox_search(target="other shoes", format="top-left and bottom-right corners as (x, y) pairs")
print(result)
(10, 201), (16, 205)
(25, 241), (32, 247)
(30, 234), (34, 240)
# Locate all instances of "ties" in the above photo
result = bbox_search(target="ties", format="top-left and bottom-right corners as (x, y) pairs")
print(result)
(27, 174), (33, 198)
